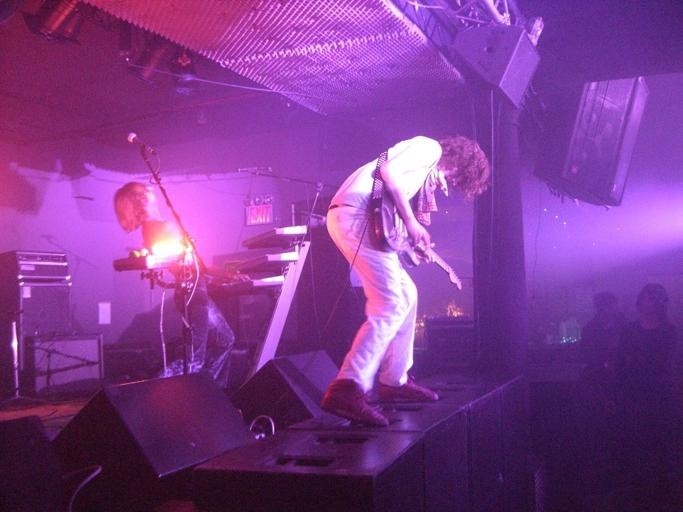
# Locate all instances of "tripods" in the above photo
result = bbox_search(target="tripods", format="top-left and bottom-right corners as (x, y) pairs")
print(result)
(0, 315), (42, 407)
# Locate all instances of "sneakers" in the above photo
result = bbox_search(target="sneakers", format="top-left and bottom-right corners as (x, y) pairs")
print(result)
(321, 379), (388, 425)
(379, 376), (440, 402)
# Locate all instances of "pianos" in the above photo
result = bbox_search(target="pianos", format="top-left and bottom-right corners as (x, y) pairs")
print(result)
(208, 273), (284, 295)
(234, 252), (301, 273)
(241, 225), (308, 247)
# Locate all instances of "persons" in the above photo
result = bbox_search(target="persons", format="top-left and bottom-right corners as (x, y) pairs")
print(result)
(603, 283), (682, 511)
(114, 183), (236, 382)
(568, 292), (629, 381)
(320, 136), (492, 427)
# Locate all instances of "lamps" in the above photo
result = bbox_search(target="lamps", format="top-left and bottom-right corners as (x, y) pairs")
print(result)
(32, 0), (77, 40)
(64, 12), (81, 39)
(128, 39), (170, 84)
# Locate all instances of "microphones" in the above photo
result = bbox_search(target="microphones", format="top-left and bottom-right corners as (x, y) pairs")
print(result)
(126, 132), (156, 155)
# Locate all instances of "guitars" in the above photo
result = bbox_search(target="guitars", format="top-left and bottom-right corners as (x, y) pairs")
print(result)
(372, 193), (462, 290)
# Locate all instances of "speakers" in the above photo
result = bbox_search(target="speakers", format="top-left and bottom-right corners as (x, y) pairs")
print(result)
(5, 281), (73, 399)
(51, 369), (258, 493)
(452, 24), (541, 109)
(0, 414), (61, 511)
(229, 349), (340, 438)
